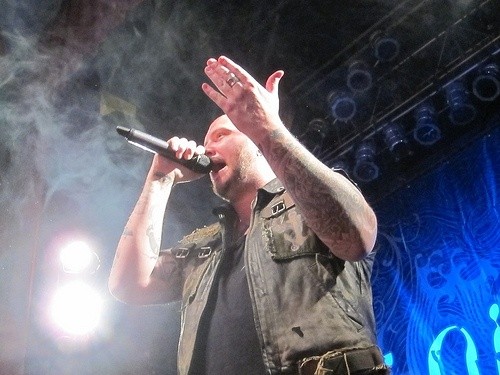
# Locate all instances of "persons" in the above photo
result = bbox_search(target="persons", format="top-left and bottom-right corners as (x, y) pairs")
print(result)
(104, 56), (397, 375)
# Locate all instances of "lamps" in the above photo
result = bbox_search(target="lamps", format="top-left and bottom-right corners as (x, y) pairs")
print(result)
(445, 82), (476, 126)
(413, 105), (441, 146)
(299, 118), (332, 155)
(473, 64), (500, 103)
(346, 60), (375, 94)
(383, 124), (412, 163)
(353, 144), (380, 183)
(369, 29), (401, 64)
(326, 90), (357, 123)
(330, 157), (353, 180)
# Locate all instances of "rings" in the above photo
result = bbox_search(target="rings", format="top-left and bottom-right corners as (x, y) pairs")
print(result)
(226, 75), (240, 87)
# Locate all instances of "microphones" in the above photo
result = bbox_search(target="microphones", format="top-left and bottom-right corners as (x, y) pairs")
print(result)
(116, 125), (213, 173)
